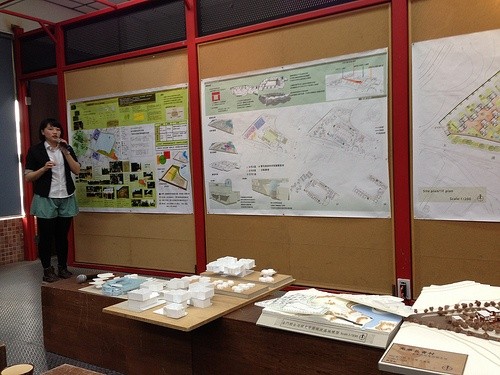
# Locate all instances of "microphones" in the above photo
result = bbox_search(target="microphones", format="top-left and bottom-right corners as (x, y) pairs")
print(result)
(76, 274), (98, 283)
(57, 139), (70, 150)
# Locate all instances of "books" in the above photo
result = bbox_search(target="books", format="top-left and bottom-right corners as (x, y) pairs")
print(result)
(254, 280), (500, 375)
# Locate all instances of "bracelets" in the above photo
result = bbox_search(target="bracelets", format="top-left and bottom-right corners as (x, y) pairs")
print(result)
(64, 152), (70, 156)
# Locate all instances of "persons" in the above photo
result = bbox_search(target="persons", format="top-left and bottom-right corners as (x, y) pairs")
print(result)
(24, 118), (80, 284)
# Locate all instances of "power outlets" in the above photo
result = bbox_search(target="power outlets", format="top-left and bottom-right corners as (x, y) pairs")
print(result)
(397, 278), (411, 300)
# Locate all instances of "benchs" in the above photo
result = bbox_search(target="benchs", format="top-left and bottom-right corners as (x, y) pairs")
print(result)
(41, 272), (400, 375)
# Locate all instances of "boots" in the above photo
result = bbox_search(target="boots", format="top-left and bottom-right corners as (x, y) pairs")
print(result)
(57, 265), (72, 279)
(43, 267), (59, 282)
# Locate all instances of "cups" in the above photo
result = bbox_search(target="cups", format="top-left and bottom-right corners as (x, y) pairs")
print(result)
(0, 364), (34, 375)
